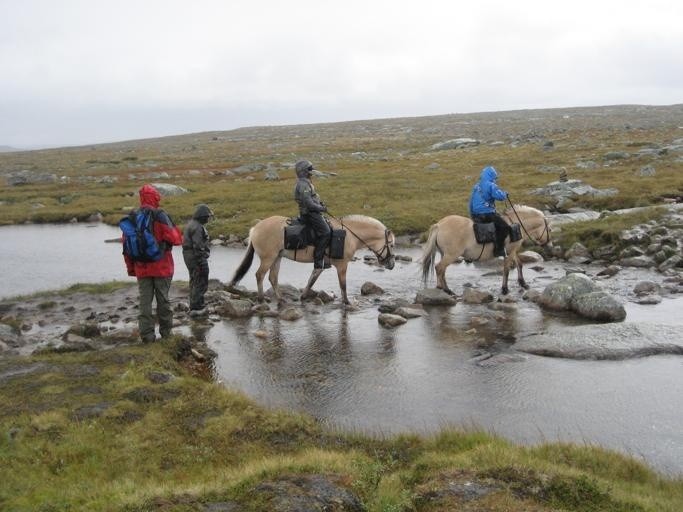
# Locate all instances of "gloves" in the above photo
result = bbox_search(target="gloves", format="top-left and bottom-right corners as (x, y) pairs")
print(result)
(320, 206), (327, 212)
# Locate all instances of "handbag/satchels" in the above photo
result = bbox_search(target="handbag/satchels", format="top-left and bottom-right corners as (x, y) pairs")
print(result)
(473, 222), (497, 245)
(509, 223), (523, 242)
(283, 225), (308, 250)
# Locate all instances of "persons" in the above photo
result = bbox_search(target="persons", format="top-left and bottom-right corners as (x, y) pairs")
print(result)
(294, 159), (331, 269)
(183, 203), (215, 316)
(469, 166), (511, 259)
(122, 183), (184, 343)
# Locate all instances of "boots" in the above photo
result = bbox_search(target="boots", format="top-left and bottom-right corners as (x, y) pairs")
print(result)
(493, 240), (510, 257)
(313, 255), (332, 269)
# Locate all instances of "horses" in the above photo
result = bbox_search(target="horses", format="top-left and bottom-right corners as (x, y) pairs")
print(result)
(227, 213), (397, 306)
(413, 204), (551, 296)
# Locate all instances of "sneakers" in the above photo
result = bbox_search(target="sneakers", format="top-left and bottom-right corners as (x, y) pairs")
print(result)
(189, 305), (208, 315)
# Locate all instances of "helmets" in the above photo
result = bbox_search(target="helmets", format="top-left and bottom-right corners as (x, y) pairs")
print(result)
(296, 160), (313, 177)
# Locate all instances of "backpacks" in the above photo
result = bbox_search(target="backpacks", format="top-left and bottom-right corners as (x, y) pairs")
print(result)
(119, 208), (164, 262)
